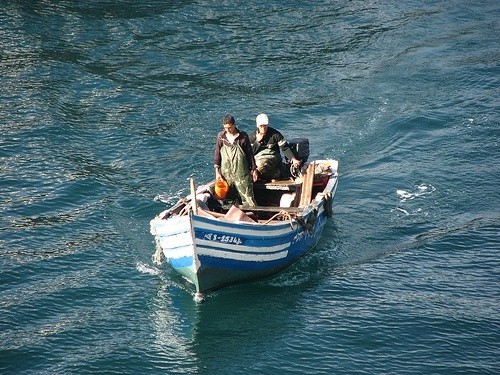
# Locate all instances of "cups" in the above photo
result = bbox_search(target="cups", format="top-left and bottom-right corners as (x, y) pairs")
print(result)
(215, 179), (229, 200)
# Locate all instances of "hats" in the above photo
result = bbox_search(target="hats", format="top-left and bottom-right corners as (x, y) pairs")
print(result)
(255, 113), (269, 128)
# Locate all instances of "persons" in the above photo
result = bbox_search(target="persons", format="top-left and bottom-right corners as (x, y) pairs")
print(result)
(244, 113), (302, 195)
(213, 115), (260, 219)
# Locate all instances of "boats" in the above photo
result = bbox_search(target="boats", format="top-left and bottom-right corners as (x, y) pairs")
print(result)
(149, 137), (339, 303)
